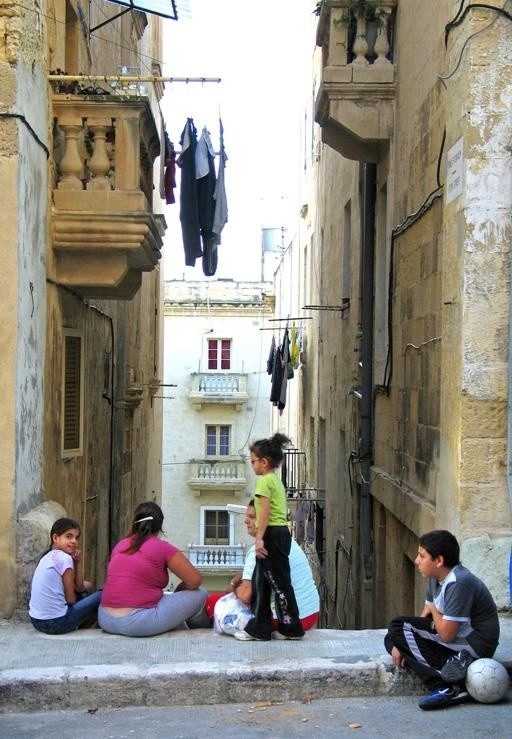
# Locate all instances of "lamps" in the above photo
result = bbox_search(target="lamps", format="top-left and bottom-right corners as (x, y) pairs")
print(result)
(126, 382), (144, 415)
(151, 377), (162, 405)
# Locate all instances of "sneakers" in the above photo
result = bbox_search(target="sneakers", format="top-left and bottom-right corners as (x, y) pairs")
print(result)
(419, 687), (469, 709)
(234, 630), (302, 641)
(441, 649), (474, 681)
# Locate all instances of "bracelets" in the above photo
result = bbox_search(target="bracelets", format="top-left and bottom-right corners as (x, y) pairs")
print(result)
(230, 579), (235, 586)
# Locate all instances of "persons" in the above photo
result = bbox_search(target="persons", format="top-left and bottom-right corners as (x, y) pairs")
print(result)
(213, 494), (322, 636)
(234, 433), (312, 642)
(384, 528), (499, 710)
(97, 500), (211, 637)
(27, 517), (102, 634)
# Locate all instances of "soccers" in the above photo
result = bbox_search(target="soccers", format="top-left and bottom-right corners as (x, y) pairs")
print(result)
(465, 657), (509, 704)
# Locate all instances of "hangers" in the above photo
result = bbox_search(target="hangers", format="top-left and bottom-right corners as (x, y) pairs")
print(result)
(78, 75), (108, 95)
